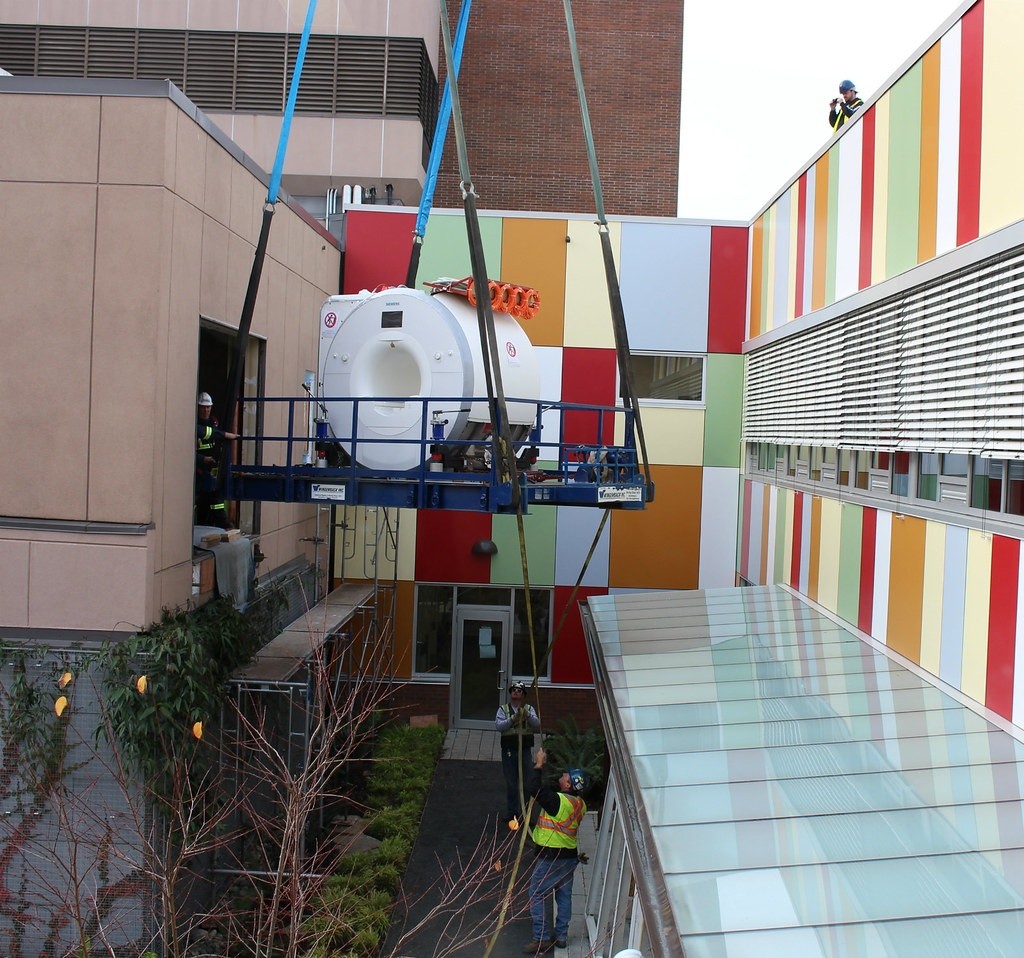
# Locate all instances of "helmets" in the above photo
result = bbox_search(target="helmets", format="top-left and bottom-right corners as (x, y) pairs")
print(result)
(839, 80), (858, 94)
(198, 391), (213, 406)
(567, 768), (592, 792)
(509, 681), (527, 696)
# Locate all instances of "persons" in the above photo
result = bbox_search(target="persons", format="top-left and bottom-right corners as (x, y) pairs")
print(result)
(829, 80), (864, 132)
(495, 682), (539, 822)
(199, 392), (240, 531)
(522, 747), (587, 953)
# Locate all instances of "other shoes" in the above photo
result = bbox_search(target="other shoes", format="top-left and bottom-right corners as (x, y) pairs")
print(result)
(522, 938), (552, 953)
(500, 815), (518, 822)
(551, 936), (566, 948)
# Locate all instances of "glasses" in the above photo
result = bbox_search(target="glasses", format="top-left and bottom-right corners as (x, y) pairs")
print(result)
(512, 688), (523, 693)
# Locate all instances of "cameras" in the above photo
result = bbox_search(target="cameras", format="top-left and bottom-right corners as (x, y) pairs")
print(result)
(835, 98), (839, 103)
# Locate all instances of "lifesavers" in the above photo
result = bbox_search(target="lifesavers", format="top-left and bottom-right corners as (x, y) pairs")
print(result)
(510, 287), (528, 318)
(468, 281), (477, 307)
(498, 284), (515, 313)
(488, 281), (503, 310)
(522, 290), (540, 319)
